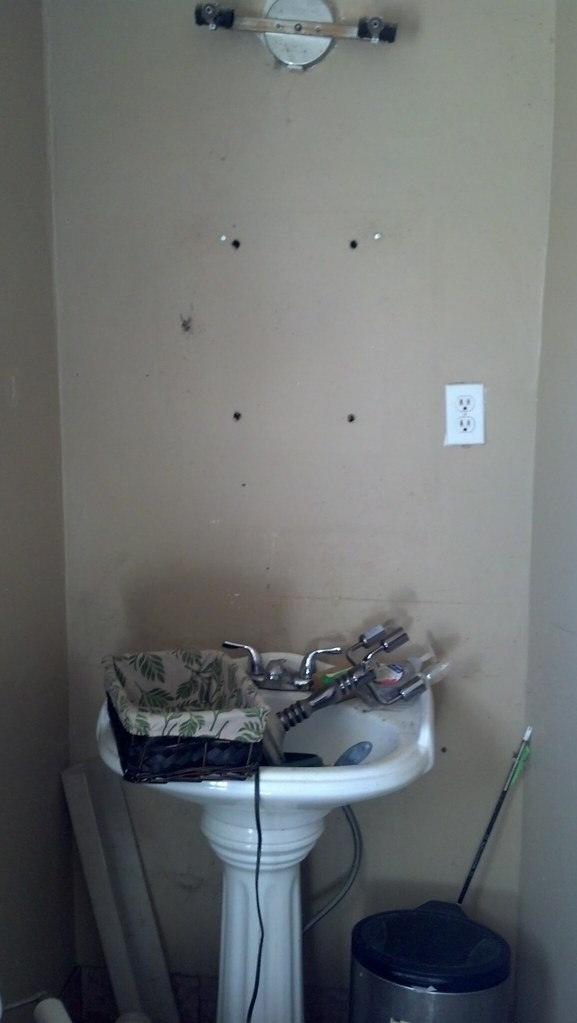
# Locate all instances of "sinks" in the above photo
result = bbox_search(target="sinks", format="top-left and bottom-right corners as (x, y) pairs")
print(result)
(95, 651), (437, 831)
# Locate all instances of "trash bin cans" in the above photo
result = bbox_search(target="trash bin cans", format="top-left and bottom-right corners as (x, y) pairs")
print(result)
(350, 899), (512, 1023)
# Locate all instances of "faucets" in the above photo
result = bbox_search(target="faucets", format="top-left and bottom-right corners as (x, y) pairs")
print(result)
(265, 659), (285, 690)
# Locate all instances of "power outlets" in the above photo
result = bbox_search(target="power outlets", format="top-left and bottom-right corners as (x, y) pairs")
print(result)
(445, 382), (485, 445)
